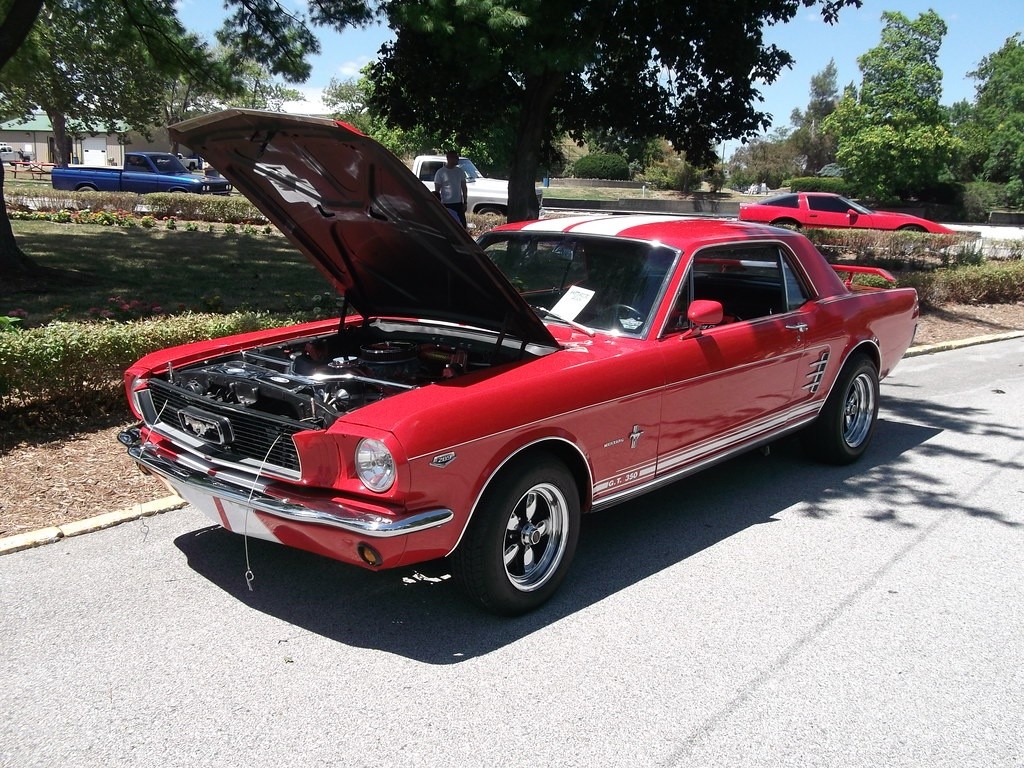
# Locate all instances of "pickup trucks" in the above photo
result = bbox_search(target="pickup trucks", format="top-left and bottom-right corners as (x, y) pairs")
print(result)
(411, 154), (544, 219)
(51, 152), (233, 198)
(1, 142), (35, 166)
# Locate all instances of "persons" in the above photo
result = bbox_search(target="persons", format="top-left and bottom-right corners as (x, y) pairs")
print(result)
(435, 150), (468, 229)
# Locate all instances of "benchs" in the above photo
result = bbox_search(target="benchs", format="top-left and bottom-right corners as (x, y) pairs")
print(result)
(6, 169), (52, 175)
(633, 274), (786, 325)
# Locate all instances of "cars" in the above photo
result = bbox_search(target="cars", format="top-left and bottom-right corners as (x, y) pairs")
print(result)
(116, 107), (919, 620)
(738, 192), (956, 236)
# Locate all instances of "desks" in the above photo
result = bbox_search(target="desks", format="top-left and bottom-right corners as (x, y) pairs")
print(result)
(10, 162), (59, 180)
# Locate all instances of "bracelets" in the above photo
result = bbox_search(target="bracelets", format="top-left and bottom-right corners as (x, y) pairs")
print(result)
(464, 202), (468, 204)
